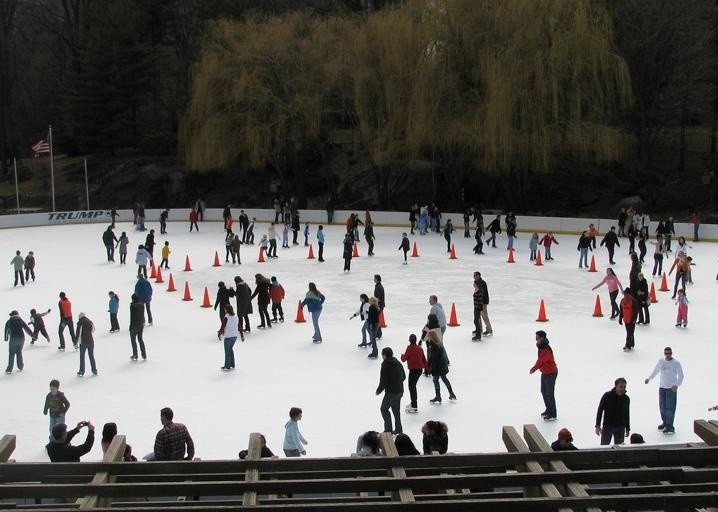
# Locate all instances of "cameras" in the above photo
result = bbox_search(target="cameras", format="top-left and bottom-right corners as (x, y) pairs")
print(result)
(83, 422), (90, 426)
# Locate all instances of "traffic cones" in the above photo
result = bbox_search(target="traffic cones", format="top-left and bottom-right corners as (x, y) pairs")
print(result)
(533, 249), (544, 265)
(659, 272), (670, 291)
(592, 295), (604, 317)
(448, 244), (457, 259)
(649, 282), (658, 303)
(536, 299), (550, 321)
(149, 242), (420, 327)
(506, 247), (515, 262)
(447, 302), (460, 327)
(587, 255), (598, 271)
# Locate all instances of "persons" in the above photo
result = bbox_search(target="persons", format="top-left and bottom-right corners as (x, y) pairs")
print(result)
(421, 420), (448, 455)
(102, 202), (174, 363)
(239, 435), (274, 459)
(213, 272), (325, 372)
(644, 346), (684, 435)
(45, 420), (95, 462)
(283, 407), (308, 457)
(395, 432), (420, 454)
(595, 377), (630, 445)
(9, 248), (37, 287)
(186, 193), (558, 271)
(43, 379), (70, 434)
(551, 428), (578, 451)
(6, 289), (98, 379)
(375, 347), (406, 436)
(101, 422), (138, 461)
(143, 407), (195, 461)
(575, 204), (701, 352)
(529, 329), (560, 422)
(355, 431), (385, 458)
(402, 331), (459, 411)
(348, 270), (496, 359)
(631, 433), (645, 444)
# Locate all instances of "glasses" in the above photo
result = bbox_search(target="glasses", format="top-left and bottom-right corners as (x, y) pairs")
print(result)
(564, 438), (573, 442)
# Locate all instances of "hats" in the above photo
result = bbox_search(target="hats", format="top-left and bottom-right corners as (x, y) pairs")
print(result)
(558, 429), (572, 440)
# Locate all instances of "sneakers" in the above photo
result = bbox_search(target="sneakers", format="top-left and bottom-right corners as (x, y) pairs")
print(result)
(243, 317), (283, 331)
(623, 344), (633, 349)
(161, 231), (167, 234)
(542, 410), (557, 419)
(392, 431), (402, 435)
(667, 249), (672, 252)
(639, 258), (644, 264)
(161, 265), (170, 269)
(411, 230), (441, 235)
(675, 323), (686, 327)
(110, 327), (119, 331)
(242, 240), (254, 244)
(636, 320), (649, 324)
(472, 331), (493, 340)
(358, 341), (376, 357)
(473, 241), (497, 254)
(657, 424), (674, 433)
(108, 257), (126, 264)
(406, 393), (457, 411)
(282, 242), (299, 247)
(268, 254), (277, 257)
(6, 368), (23, 372)
(130, 356), (146, 359)
(368, 252), (375, 256)
(578, 264), (589, 268)
(318, 258), (324, 261)
(221, 365), (234, 369)
(610, 311), (619, 319)
(30, 339), (97, 375)
(610, 261), (615, 264)
(530, 257), (554, 260)
(226, 260), (241, 264)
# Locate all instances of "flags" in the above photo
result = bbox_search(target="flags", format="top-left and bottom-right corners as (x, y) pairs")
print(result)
(31, 132), (51, 153)
